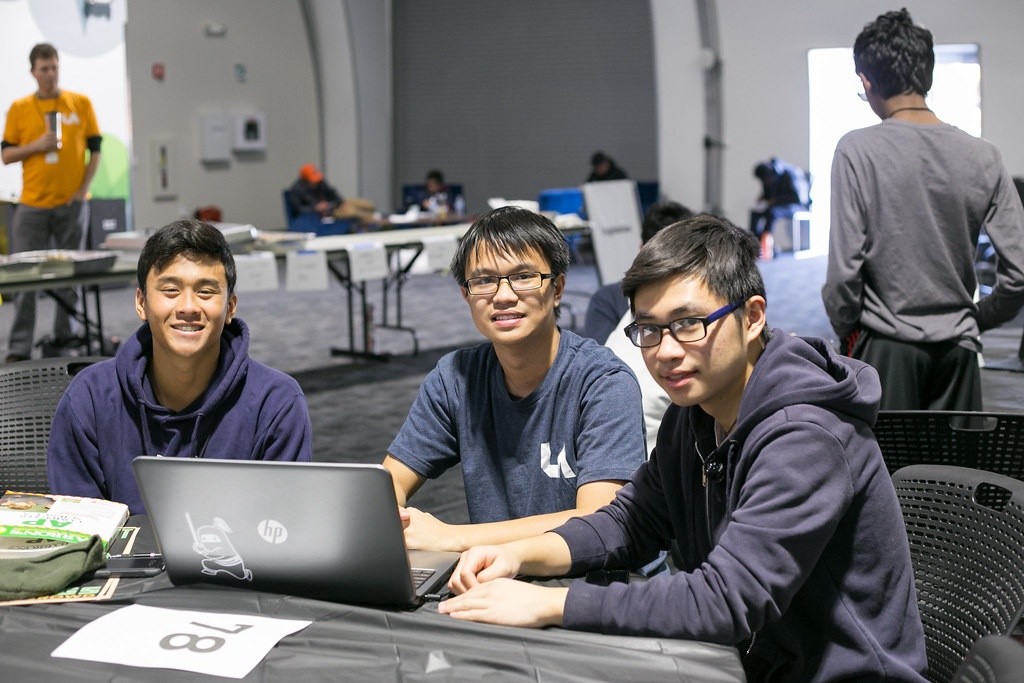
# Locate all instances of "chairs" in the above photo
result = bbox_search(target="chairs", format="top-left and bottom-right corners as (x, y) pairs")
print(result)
(0, 353), (114, 497)
(873, 407), (1024, 508)
(790, 171), (815, 258)
(888, 465), (1024, 683)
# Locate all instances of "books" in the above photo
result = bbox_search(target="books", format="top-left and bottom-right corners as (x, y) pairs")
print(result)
(0, 490), (130, 561)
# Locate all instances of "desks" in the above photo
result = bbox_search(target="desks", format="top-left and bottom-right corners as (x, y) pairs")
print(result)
(1, 216), (591, 363)
(1, 573), (746, 682)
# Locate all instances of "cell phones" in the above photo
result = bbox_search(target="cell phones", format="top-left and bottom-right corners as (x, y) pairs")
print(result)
(95, 554), (165, 578)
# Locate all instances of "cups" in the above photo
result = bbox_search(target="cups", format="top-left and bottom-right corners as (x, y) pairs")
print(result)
(46, 111), (62, 142)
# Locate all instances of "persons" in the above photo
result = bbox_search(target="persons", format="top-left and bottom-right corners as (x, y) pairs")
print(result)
(337, 170), (451, 225)
(748, 157), (809, 254)
(588, 150), (627, 181)
(0, 43), (102, 363)
(290, 164), (343, 218)
(581, 201), (696, 456)
(436, 213), (929, 683)
(820, 6), (1024, 411)
(382, 206), (647, 553)
(47, 217), (313, 517)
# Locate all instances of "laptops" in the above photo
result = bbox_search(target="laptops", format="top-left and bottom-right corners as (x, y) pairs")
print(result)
(131, 455), (463, 609)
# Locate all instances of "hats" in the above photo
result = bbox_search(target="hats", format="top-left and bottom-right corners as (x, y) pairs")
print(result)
(300, 164), (321, 183)
(0, 534), (107, 602)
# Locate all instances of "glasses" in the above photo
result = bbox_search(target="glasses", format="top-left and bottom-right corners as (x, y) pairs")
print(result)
(463, 271), (557, 296)
(623, 296), (749, 348)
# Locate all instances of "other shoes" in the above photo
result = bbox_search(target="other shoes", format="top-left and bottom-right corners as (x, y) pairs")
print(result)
(6, 354), (30, 364)
